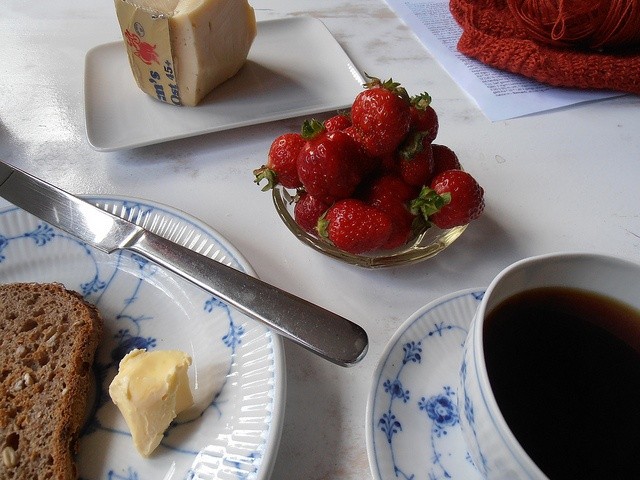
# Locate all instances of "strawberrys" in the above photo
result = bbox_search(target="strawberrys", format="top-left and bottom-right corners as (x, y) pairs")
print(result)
(252, 70), (486, 255)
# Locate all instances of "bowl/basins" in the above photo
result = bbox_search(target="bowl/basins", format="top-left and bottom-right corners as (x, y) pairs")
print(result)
(457, 252), (639, 480)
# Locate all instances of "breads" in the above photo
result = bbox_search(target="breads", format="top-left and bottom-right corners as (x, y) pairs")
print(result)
(0, 281), (100, 479)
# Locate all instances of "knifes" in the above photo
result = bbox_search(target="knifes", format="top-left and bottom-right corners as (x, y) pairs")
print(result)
(0, 162), (367, 368)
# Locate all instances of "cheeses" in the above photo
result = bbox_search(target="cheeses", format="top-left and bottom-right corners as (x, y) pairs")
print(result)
(169, 0), (259, 108)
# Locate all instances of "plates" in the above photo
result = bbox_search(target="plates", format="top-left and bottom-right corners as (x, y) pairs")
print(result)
(365, 286), (485, 480)
(268, 183), (468, 267)
(0, 195), (286, 480)
(83, 15), (366, 153)
